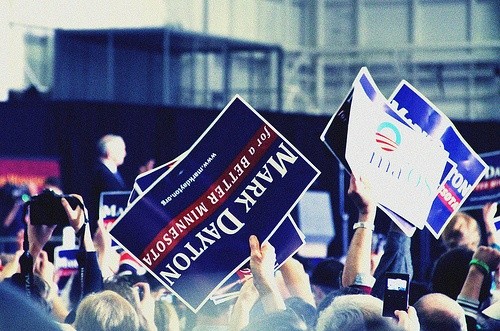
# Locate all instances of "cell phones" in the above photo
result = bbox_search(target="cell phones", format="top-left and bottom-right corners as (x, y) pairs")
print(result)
(382, 273), (411, 317)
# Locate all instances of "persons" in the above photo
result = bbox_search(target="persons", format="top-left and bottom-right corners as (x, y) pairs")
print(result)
(0, 134), (500, 331)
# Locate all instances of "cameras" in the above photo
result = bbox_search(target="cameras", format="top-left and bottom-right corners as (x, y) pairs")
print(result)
(30, 188), (79, 227)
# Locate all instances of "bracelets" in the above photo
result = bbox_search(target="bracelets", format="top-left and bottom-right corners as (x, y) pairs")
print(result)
(352, 222), (375, 232)
(486, 231), (496, 237)
(468, 259), (490, 276)
